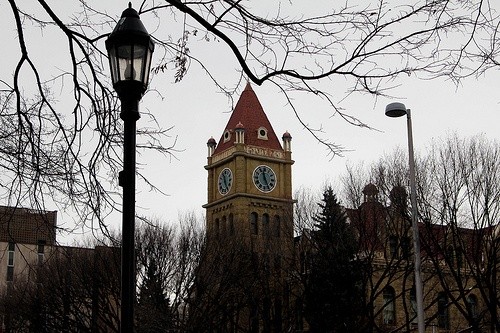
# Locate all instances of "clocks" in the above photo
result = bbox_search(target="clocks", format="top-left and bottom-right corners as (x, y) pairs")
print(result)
(252, 165), (277, 193)
(218, 168), (233, 196)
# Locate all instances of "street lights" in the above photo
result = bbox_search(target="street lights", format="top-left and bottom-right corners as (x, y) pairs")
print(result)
(102, 2), (154, 332)
(385, 102), (428, 332)
